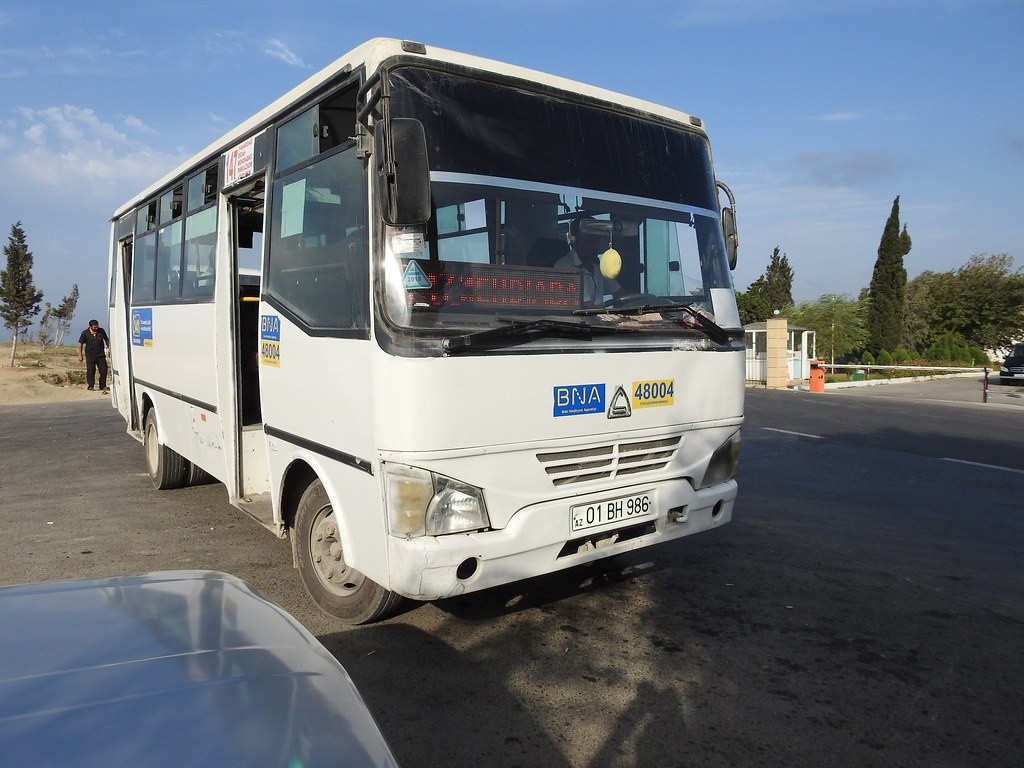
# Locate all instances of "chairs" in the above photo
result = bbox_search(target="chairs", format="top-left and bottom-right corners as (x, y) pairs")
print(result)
(169, 271), (259, 417)
(531, 240), (571, 268)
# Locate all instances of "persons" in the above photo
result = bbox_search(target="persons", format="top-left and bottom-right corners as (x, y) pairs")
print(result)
(79, 320), (110, 391)
(554, 215), (632, 308)
(194, 247), (216, 287)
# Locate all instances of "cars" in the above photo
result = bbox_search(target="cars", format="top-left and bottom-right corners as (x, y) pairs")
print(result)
(998, 343), (1024, 386)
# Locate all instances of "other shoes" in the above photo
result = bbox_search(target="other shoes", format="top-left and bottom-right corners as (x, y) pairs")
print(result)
(101, 386), (110, 390)
(87, 385), (94, 390)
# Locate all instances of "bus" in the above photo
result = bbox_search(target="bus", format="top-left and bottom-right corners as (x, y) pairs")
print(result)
(108, 36), (747, 626)
